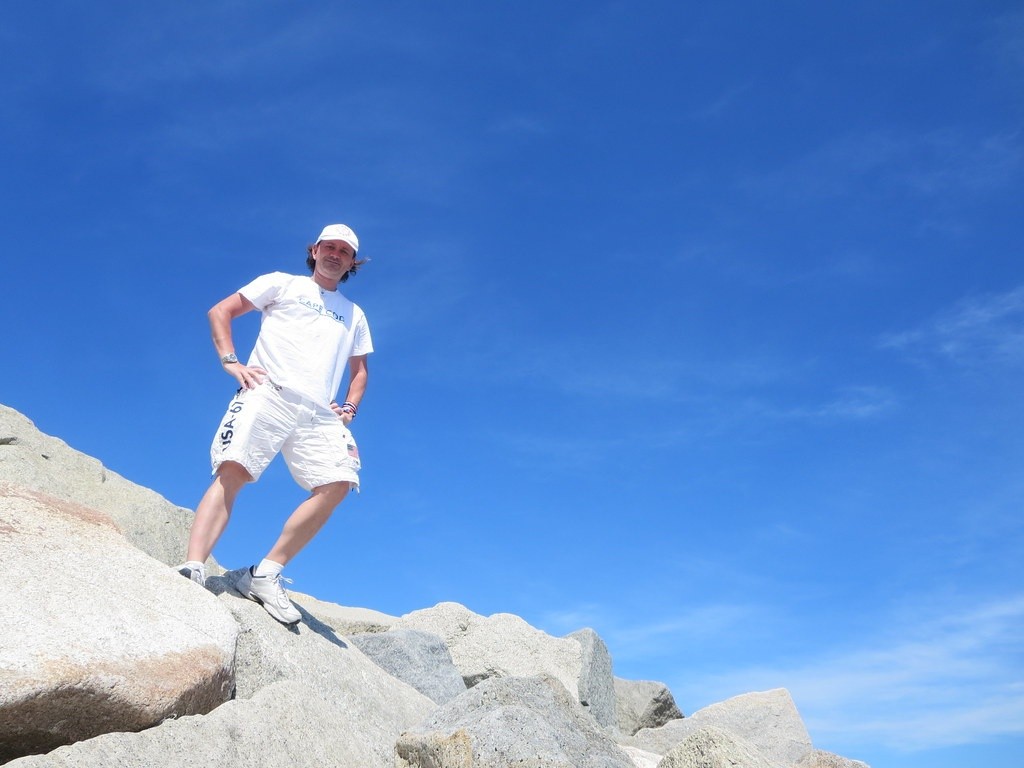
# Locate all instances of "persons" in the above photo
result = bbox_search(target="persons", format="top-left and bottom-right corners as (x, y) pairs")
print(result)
(178, 224), (374, 624)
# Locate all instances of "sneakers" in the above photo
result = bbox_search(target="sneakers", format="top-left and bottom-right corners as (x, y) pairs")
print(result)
(177, 561), (206, 587)
(235, 565), (302, 625)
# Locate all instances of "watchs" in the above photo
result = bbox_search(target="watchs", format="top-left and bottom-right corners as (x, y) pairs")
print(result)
(220, 352), (238, 366)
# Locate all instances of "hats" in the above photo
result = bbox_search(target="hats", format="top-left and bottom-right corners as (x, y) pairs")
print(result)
(316, 223), (359, 258)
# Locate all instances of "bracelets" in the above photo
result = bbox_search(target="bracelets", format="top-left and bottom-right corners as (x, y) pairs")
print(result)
(342, 402), (358, 417)
